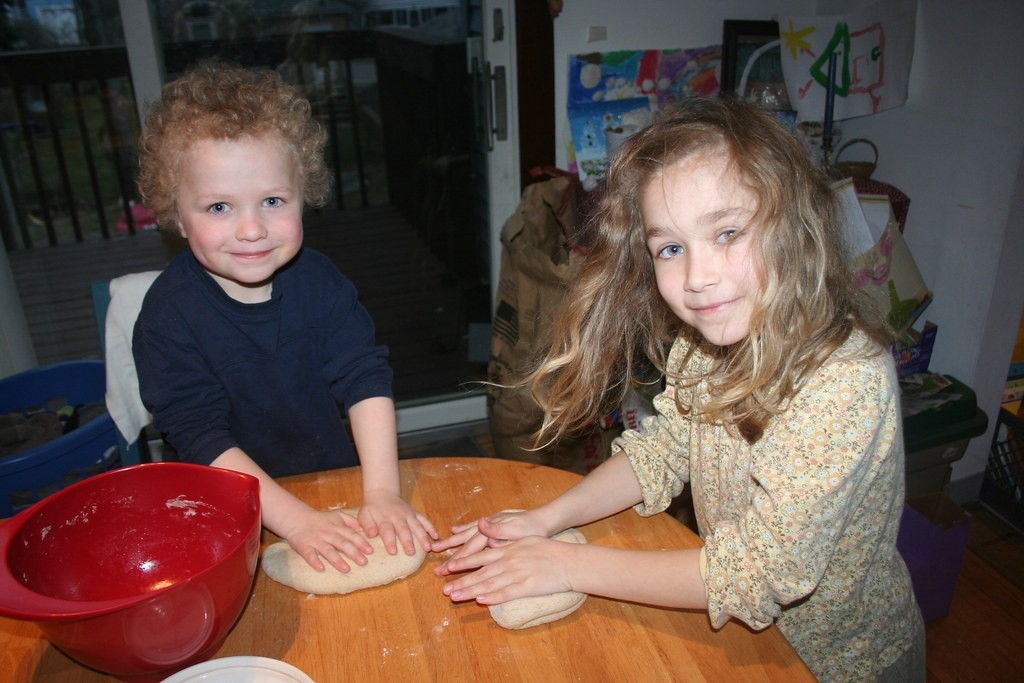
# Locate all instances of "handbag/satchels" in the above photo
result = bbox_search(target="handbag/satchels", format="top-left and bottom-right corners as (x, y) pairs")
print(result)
(840, 193), (933, 341)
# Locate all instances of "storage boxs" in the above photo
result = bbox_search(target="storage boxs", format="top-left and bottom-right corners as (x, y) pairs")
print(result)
(976, 399), (1024, 550)
(895, 371), (988, 497)
(886, 318), (938, 382)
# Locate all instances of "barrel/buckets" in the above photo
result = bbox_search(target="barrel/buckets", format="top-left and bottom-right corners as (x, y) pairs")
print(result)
(0, 361), (123, 518)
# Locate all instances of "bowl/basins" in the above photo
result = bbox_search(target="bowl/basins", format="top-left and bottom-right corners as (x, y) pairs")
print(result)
(0, 463), (260, 683)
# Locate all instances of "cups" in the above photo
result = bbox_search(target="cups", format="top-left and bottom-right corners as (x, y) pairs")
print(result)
(604, 125), (640, 153)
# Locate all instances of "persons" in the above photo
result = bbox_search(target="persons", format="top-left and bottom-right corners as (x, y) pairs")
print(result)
(433, 102), (926, 683)
(131, 59), (439, 575)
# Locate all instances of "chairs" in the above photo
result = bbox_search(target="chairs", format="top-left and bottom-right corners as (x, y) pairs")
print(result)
(95, 269), (177, 466)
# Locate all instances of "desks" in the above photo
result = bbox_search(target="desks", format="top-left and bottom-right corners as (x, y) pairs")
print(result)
(0, 457), (819, 682)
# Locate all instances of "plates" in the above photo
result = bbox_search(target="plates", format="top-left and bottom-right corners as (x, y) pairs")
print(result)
(158, 656), (316, 683)
